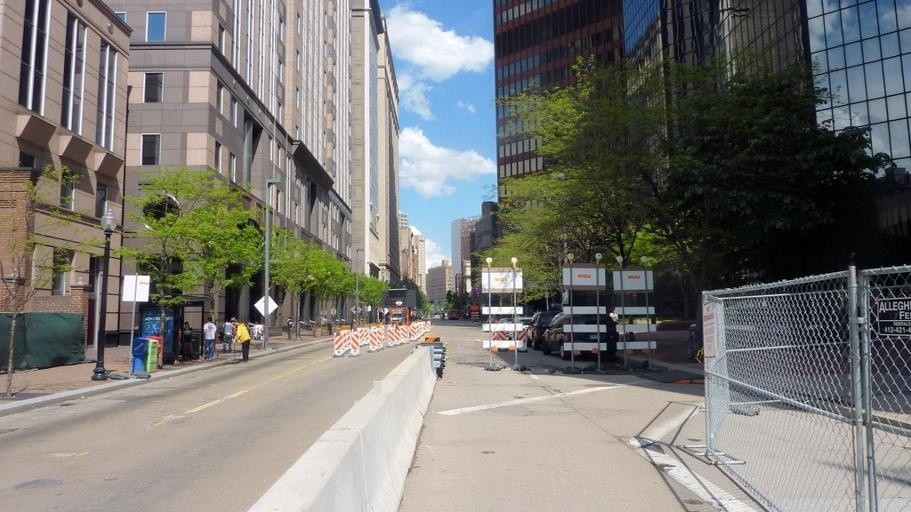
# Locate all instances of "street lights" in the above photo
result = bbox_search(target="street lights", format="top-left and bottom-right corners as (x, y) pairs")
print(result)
(90, 209), (117, 381)
(551, 169), (568, 266)
(263, 179), (280, 350)
(355, 247), (364, 324)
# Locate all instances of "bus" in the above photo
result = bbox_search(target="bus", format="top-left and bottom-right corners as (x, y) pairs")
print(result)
(383, 289), (417, 327)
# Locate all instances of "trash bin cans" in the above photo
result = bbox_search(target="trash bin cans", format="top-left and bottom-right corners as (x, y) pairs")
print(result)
(183, 331), (200, 361)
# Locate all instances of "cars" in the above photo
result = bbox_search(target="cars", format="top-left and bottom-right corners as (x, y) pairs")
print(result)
(483, 308), (619, 362)
(687, 302), (788, 361)
(421, 311), (448, 320)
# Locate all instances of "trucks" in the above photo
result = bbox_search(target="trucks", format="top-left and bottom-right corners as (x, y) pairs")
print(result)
(448, 309), (460, 320)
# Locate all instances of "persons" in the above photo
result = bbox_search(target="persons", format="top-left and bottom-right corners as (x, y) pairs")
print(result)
(287, 317), (294, 340)
(183, 321), (193, 359)
(203, 317), (217, 361)
(234, 323), (250, 361)
(223, 318), (235, 351)
